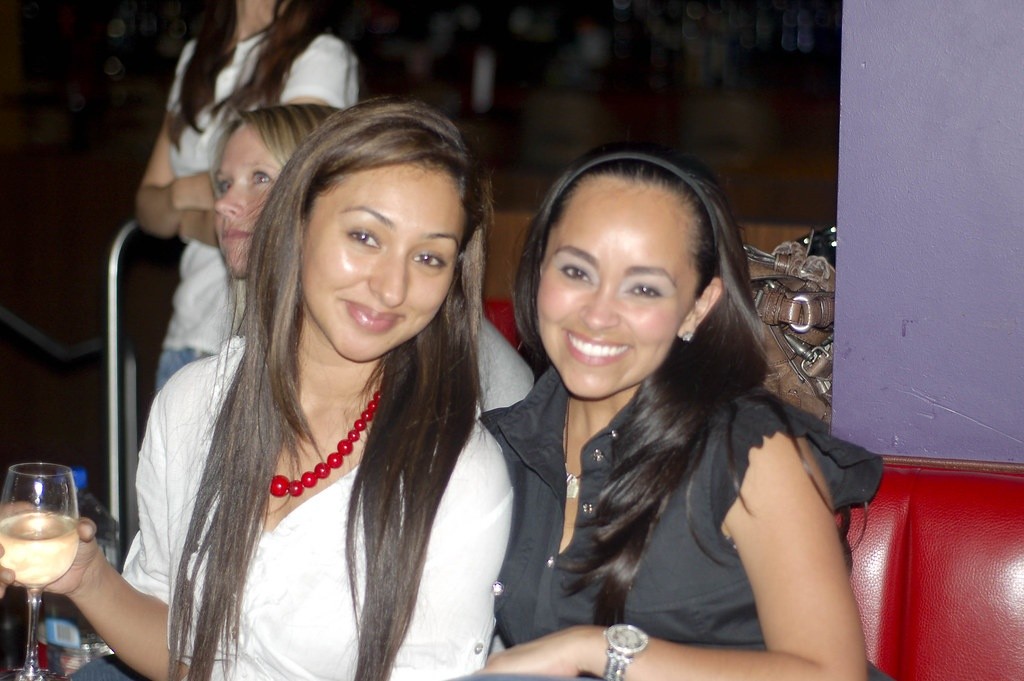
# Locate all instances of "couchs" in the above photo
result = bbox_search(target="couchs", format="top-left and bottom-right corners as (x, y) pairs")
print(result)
(837, 456), (1024, 680)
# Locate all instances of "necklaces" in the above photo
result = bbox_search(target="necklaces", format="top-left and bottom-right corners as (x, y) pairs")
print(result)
(271, 389), (384, 500)
(559, 398), (595, 498)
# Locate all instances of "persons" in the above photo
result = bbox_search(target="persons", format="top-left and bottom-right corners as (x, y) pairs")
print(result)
(128, 1), (363, 401)
(211, 98), (535, 418)
(2, 4), (840, 140)
(0, 103), (515, 681)
(473, 142), (885, 681)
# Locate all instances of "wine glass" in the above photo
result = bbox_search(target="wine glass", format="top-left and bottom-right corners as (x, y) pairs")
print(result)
(0, 461), (81, 681)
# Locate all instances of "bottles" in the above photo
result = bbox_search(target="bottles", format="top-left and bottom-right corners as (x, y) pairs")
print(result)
(41, 465), (121, 676)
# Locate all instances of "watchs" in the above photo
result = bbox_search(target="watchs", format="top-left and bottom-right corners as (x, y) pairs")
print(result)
(600, 621), (649, 681)
